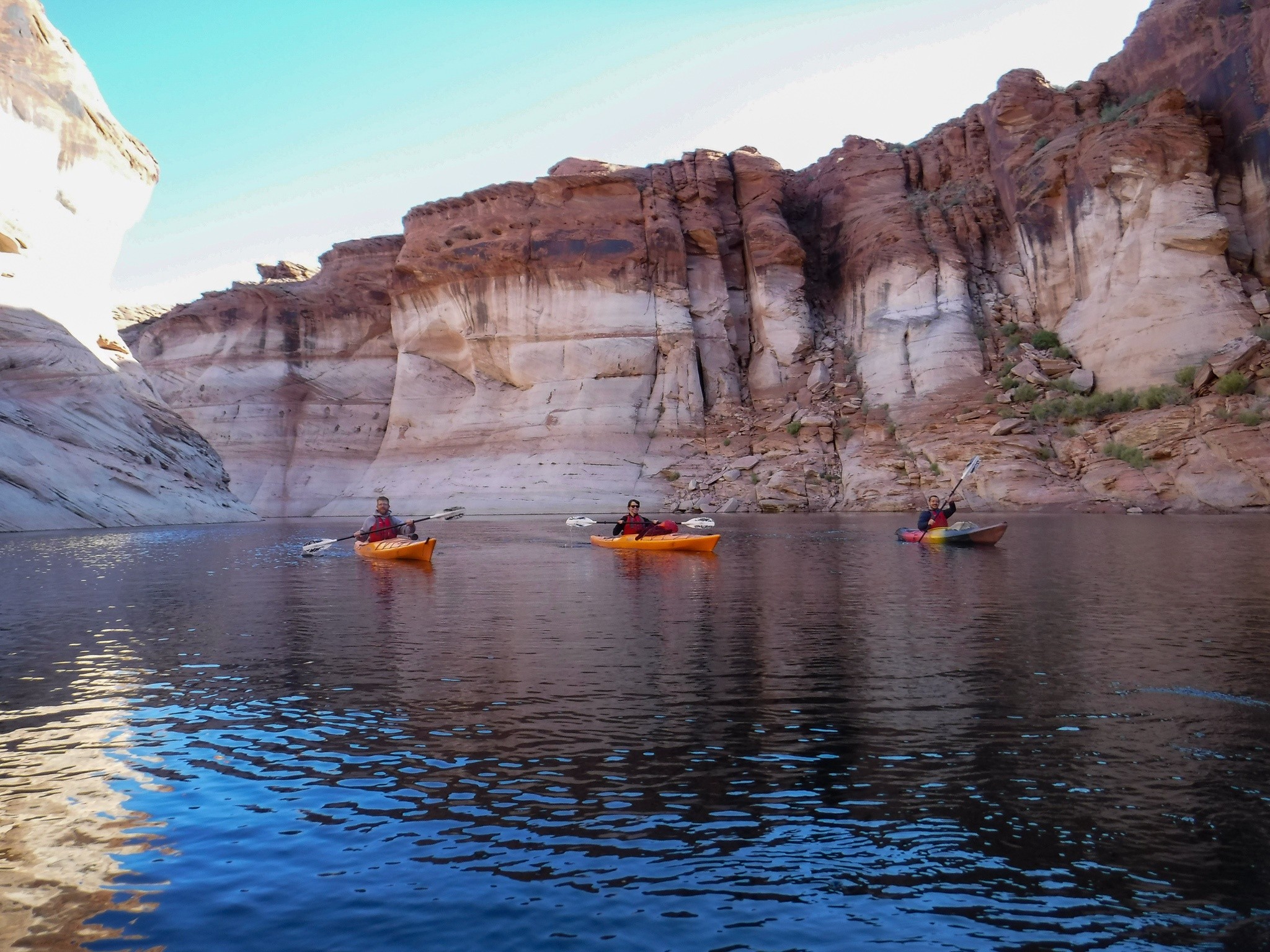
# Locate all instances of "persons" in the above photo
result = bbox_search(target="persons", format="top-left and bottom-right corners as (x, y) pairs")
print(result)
(613, 499), (659, 537)
(917, 495), (957, 530)
(353, 496), (416, 542)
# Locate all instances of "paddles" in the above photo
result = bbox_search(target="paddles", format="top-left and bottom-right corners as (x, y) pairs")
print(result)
(565, 515), (716, 530)
(302, 504), (468, 557)
(918, 451), (983, 547)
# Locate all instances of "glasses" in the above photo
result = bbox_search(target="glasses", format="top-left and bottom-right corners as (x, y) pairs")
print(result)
(631, 505), (639, 508)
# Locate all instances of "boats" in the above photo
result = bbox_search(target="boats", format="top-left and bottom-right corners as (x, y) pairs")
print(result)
(895, 520), (1008, 546)
(354, 536), (437, 563)
(589, 533), (721, 552)
